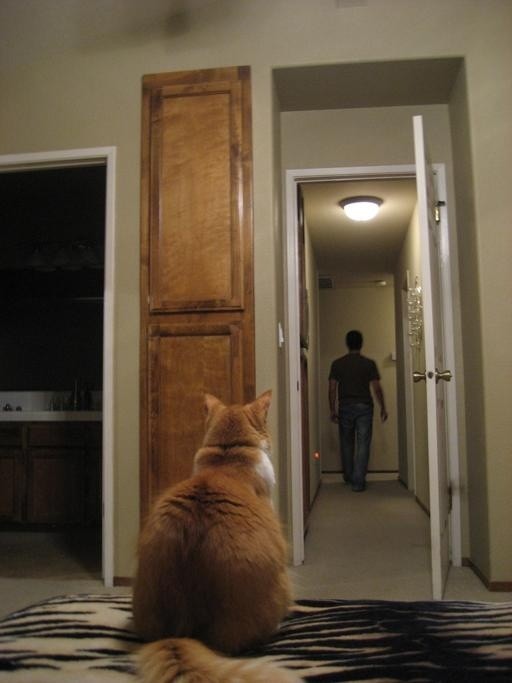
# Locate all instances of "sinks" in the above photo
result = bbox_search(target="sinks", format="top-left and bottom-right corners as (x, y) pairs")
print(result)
(0, 389), (103, 423)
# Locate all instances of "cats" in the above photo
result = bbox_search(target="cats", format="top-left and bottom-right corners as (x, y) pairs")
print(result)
(124, 389), (306, 683)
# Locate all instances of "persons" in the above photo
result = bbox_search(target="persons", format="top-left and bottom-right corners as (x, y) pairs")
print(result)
(329, 330), (388, 492)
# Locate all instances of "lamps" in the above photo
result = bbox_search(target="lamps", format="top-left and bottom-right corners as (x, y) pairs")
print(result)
(337, 195), (384, 222)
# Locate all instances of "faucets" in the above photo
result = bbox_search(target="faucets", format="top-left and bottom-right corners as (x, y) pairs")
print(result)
(72, 375), (81, 408)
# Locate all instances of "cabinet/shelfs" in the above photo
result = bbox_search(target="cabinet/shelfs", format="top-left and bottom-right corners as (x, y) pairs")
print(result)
(134, 64), (256, 535)
(1, 423), (101, 528)
